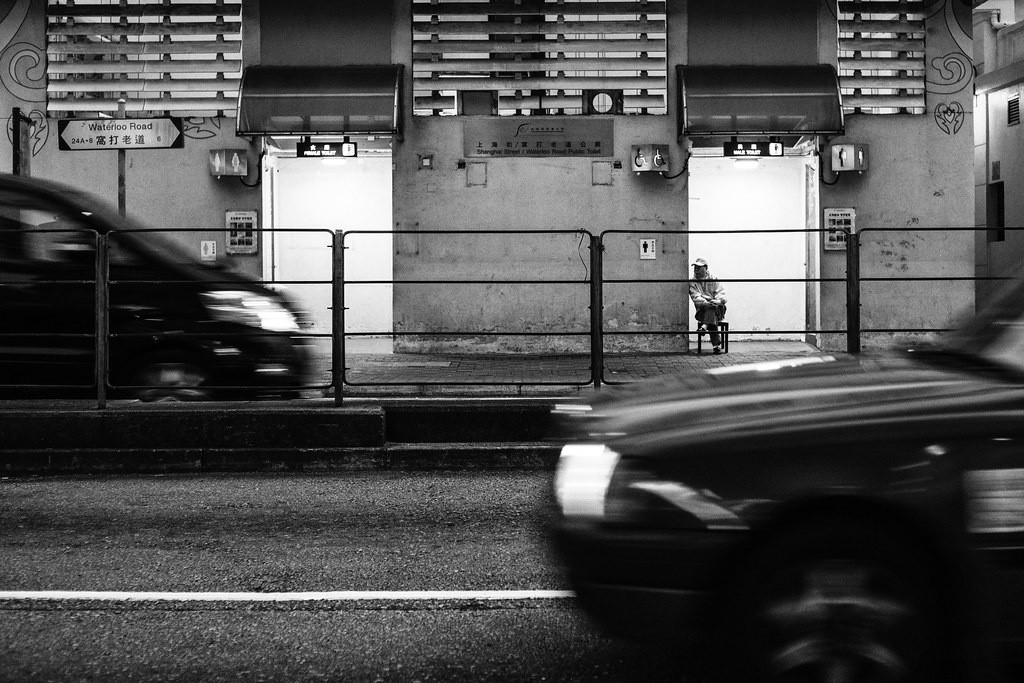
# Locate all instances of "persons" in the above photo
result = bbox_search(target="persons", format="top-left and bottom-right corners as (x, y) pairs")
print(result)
(689, 258), (728, 355)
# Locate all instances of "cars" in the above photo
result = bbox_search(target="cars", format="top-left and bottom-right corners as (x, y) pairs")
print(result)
(536, 255), (1023, 683)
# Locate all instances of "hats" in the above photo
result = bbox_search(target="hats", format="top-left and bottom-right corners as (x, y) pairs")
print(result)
(691, 258), (709, 266)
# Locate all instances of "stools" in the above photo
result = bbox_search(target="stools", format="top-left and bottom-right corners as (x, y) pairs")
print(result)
(698, 321), (729, 353)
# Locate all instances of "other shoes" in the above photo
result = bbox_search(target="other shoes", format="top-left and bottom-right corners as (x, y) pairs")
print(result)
(696, 327), (706, 336)
(713, 347), (720, 353)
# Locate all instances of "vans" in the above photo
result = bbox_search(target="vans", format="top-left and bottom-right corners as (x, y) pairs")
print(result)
(0, 171), (312, 401)
